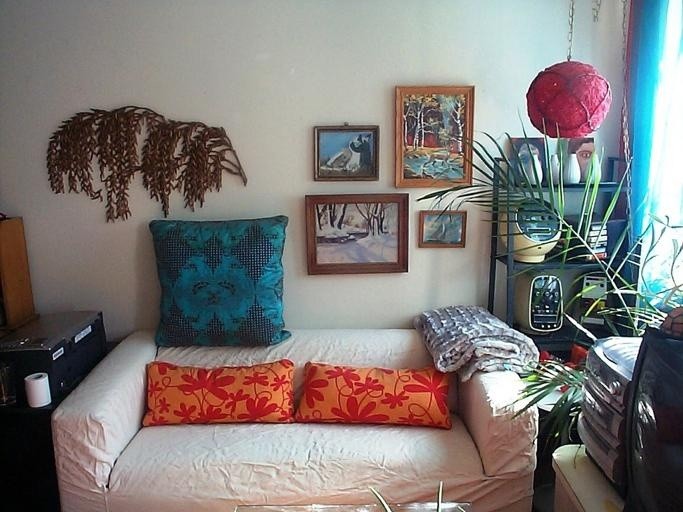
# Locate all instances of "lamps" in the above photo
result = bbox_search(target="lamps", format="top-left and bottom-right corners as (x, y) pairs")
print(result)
(525, 1), (612, 141)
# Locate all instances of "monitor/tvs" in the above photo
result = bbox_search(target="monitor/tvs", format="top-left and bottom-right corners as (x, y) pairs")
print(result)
(626, 326), (683, 512)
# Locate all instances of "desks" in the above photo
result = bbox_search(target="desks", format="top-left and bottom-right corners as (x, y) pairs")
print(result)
(1, 310), (104, 509)
(533, 381), (582, 511)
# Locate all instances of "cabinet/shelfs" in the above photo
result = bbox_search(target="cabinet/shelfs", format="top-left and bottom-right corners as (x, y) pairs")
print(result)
(488, 154), (632, 361)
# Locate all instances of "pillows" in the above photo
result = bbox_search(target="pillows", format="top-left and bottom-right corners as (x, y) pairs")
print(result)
(147, 215), (292, 345)
(142, 359), (451, 430)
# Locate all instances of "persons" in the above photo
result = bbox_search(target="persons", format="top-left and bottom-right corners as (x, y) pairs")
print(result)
(512, 138), (546, 187)
(567, 139), (594, 181)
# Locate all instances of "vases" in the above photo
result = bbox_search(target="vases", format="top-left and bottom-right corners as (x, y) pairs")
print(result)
(524, 152), (582, 186)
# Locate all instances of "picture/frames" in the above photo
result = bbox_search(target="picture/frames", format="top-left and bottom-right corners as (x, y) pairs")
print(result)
(301, 83), (475, 279)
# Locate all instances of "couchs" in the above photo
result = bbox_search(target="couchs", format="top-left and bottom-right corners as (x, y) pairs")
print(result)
(45, 324), (540, 510)
